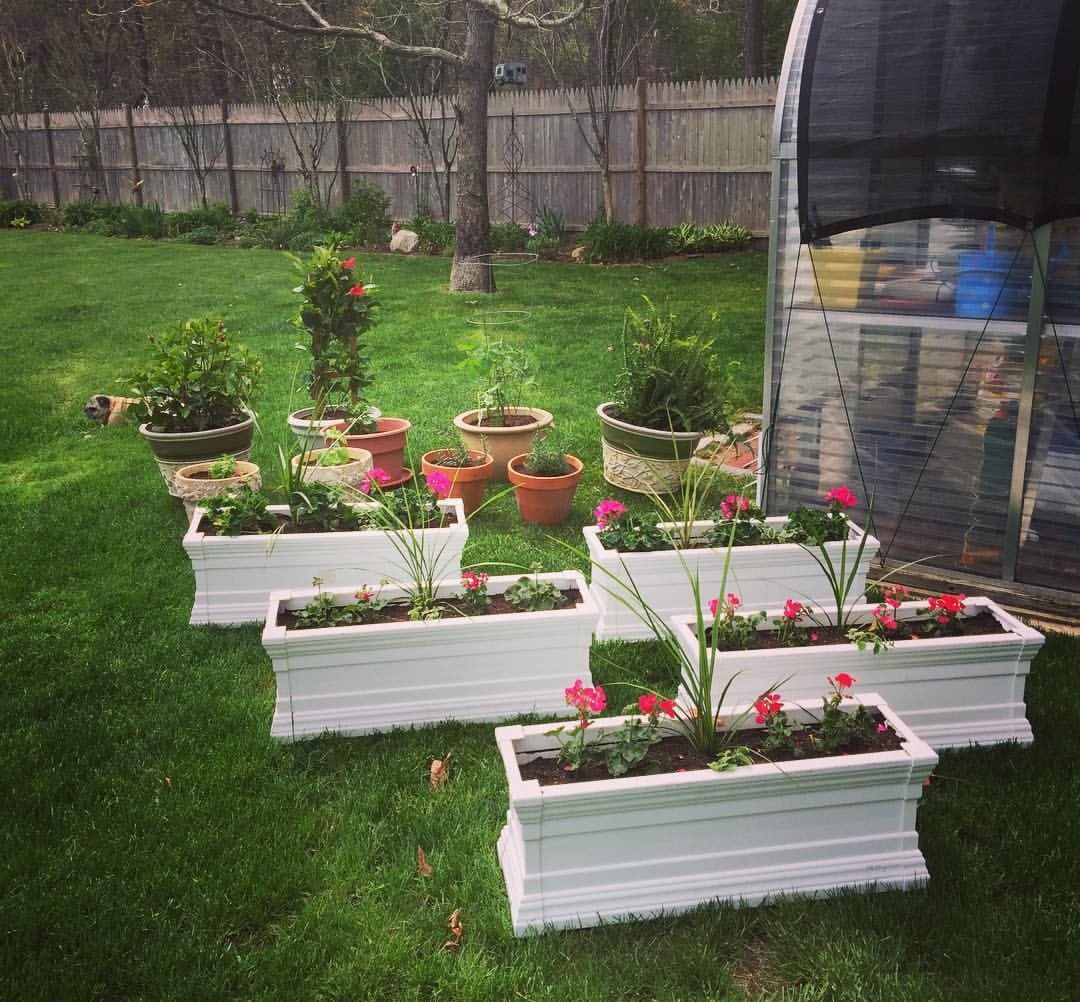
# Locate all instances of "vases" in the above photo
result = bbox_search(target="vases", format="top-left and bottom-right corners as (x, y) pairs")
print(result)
(321, 416), (413, 492)
(258, 567), (597, 744)
(498, 687), (938, 939)
(138, 407), (257, 498)
(182, 495), (469, 625)
(669, 594), (1046, 748)
(582, 509), (881, 643)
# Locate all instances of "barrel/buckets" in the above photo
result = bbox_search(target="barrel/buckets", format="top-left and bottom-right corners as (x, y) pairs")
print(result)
(956, 252), (1028, 321)
(809, 246), (883, 309)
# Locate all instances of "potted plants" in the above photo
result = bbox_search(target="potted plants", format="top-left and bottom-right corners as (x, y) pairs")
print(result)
(172, 452), (262, 527)
(421, 423), (494, 516)
(274, 241), (383, 451)
(453, 250), (553, 484)
(507, 418), (584, 526)
(288, 425), (373, 503)
(596, 288), (760, 493)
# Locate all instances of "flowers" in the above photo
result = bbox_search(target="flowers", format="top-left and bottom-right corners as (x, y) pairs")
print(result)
(116, 246), (969, 788)
